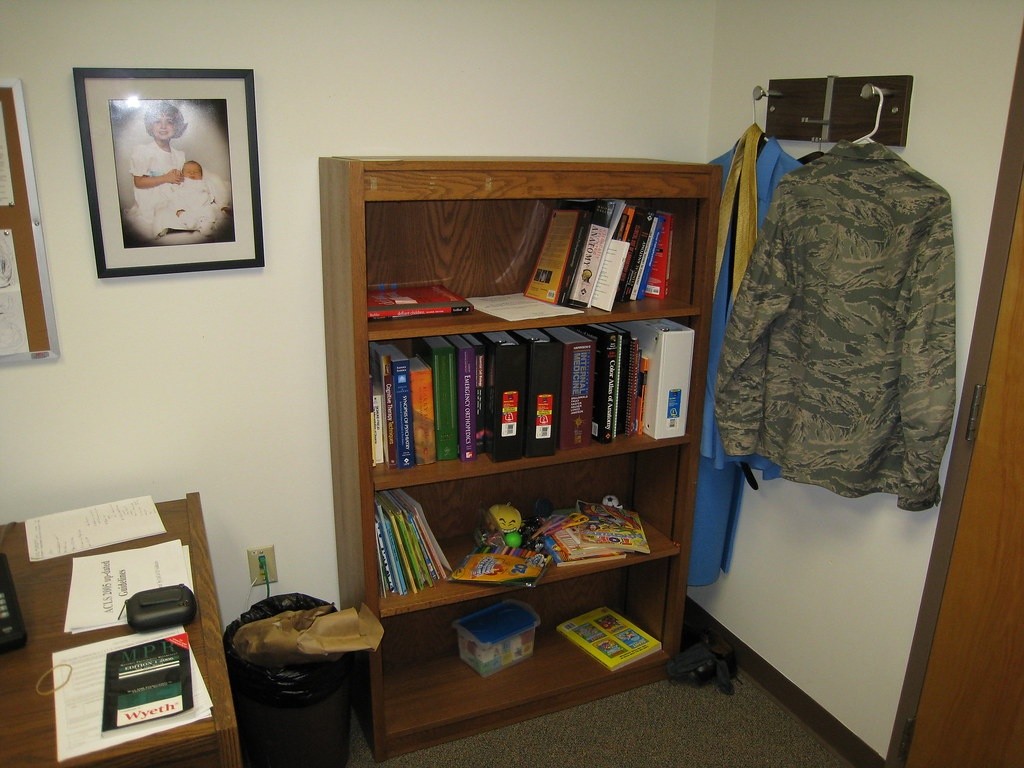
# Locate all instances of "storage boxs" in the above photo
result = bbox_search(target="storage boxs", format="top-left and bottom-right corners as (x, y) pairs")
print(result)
(451, 599), (541, 678)
(612, 318), (695, 440)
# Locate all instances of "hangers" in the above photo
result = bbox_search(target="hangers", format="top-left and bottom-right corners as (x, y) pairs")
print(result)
(850, 86), (884, 145)
(752, 90), (770, 142)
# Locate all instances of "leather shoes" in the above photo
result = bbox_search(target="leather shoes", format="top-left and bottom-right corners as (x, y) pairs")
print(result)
(683, 621), (737, 684)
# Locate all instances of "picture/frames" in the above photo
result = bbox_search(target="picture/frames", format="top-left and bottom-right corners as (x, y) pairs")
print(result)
(72, 66), (266, 279)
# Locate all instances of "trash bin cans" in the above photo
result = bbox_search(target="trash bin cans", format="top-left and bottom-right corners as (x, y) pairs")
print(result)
(227, 593), (353, 768)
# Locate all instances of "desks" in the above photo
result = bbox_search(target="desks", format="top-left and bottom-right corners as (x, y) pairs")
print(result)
(0, 490), (243, 768)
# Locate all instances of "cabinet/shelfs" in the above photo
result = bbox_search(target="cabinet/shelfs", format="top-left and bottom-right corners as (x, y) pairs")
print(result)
(318, 156), (723, 763)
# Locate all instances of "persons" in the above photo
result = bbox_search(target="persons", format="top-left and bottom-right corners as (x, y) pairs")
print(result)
(127, 102), (233, 240)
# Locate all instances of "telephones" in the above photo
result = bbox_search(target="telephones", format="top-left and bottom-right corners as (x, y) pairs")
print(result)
(0, 551), (29, 652)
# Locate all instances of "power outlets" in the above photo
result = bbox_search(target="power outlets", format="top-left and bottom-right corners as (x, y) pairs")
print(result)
(247, 545), (279, 586)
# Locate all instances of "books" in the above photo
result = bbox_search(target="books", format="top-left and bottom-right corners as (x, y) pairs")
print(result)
(544, 499), (651, 568)
(101, 632), (197, 739)
(367, 284), (474, 321)
(523, 200), (675, 312)
(369, 323), (648, 469)
(374, 488), (455, 599)
(556, 605), (662, 671)
